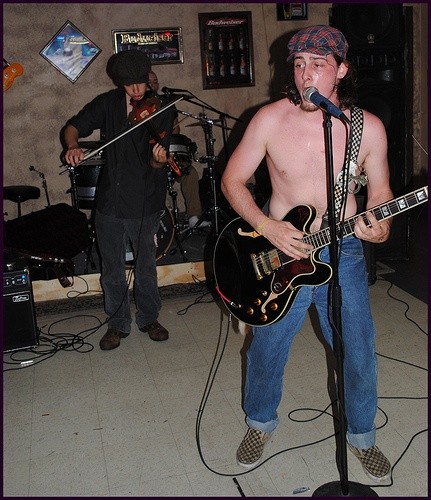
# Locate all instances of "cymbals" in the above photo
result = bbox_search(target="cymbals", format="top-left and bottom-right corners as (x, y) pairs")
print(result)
(160, 94), (195, 100)
(185, 119), (221, 127)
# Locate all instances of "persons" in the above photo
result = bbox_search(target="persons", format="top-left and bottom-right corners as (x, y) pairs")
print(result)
(219, 21), (397, 478)
(146, 69), (213, 229)
(61, 48), (176, 350)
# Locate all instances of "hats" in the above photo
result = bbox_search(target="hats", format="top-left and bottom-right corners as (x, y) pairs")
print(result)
(111, 49), (150, 84)
(286, 25), (349, 64)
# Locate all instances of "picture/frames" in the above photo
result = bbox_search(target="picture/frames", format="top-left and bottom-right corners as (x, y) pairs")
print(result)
(39, 19), (103, 84)
(111, 27), (184, 65)
(198, 10), (256, 90)
(277, 2), (308, 21)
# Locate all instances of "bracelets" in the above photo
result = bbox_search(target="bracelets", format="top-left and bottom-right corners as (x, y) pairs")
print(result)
(259, 218), (271, 236)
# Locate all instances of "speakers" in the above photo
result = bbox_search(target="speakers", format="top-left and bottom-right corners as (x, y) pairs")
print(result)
(354, 186), (376, 282)
(329, 1), (414, 260)
(3, 265), (39, 353)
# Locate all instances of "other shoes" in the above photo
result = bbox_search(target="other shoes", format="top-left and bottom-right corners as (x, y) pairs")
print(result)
(138, 322), (169, 341)
(235, 427), (277, 470)
(98, 329), (123, 351)
(347, 443), (392, 479)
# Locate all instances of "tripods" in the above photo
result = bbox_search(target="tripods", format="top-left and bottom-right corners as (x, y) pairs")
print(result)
(176, 110), (233, 236)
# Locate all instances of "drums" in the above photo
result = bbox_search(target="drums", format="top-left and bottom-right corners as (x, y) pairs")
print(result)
(71, 151), (104, 201)
(166, 134), (192, 171)
(122, 200), (175, 268)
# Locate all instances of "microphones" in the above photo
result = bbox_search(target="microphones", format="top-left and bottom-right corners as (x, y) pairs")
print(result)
(303, 86), (353, 123)
(161, 86), (188, 93)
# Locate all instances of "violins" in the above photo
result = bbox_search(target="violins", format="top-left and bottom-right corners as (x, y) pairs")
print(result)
(128, 92), (183, 176)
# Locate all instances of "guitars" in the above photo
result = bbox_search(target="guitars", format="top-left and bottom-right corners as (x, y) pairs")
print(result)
(212, 185), (428, 329)
